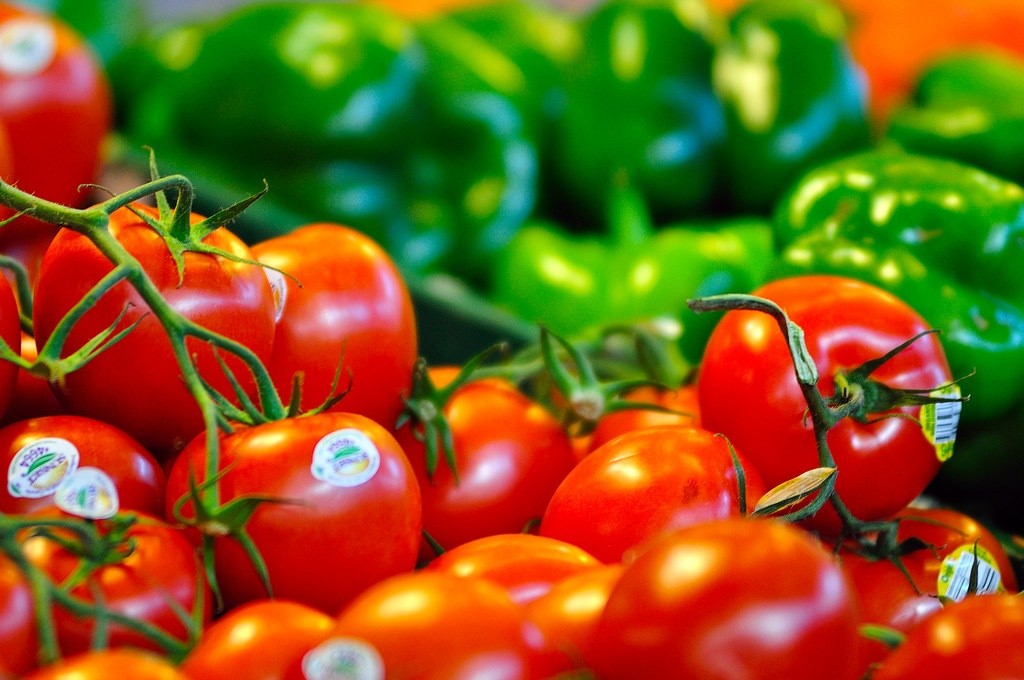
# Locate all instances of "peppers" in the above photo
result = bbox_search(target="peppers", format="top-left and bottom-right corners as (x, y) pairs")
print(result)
(26, 0), (1024, 534)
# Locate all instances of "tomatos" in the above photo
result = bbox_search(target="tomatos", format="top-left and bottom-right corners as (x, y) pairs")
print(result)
(0, 0), (108, 238)
(0, 173), (1024, 680)
